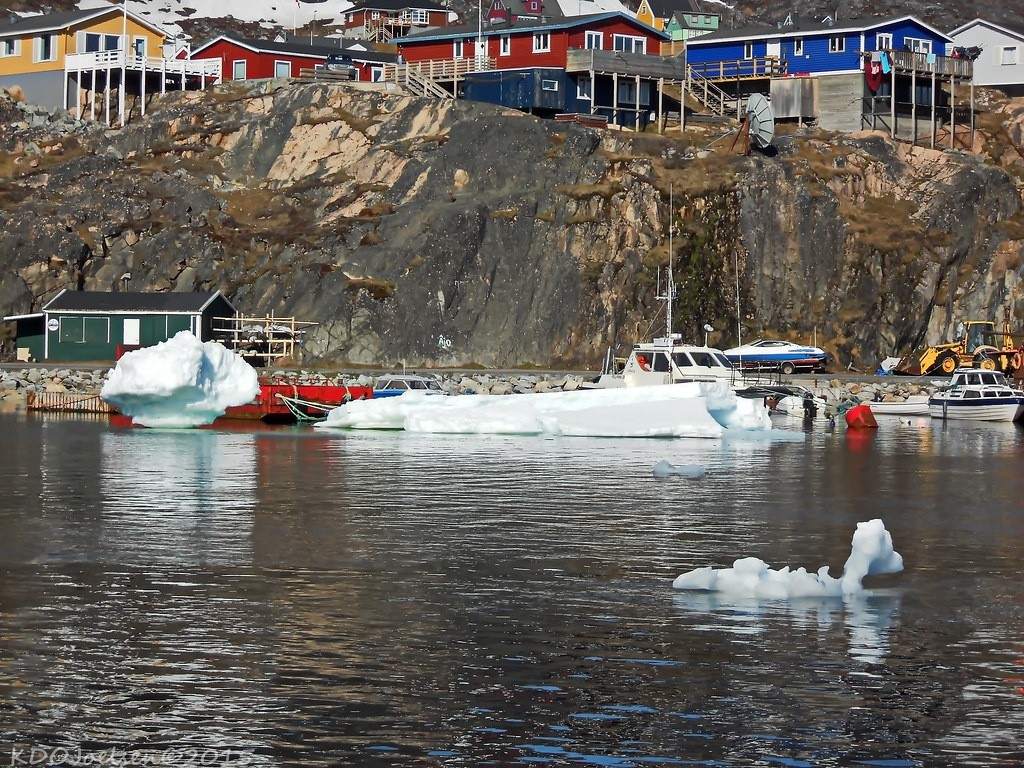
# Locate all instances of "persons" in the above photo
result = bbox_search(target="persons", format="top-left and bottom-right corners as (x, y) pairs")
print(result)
(637, 354), (652, 372)
(902, 44), (911, 53)
(951, 46), (961, 58)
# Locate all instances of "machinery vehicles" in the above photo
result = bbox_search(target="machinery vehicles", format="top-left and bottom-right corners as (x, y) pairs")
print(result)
(892, 305), (1021, 378)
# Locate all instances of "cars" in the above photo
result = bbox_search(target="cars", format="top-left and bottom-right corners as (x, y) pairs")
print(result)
(324, 53), (356, 80)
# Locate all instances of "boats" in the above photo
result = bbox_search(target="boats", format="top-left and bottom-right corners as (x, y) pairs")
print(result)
(928, 361), (1024, 422)
(860, 401), (930, 415)
(776, 385), (827, 408)
(578, 183), (794, 417)
(722, 339), (827, 368)
(373, 375), (450, 399)
